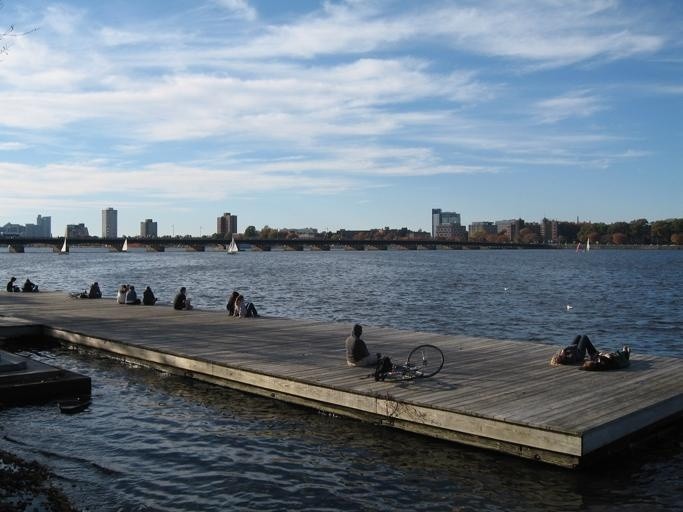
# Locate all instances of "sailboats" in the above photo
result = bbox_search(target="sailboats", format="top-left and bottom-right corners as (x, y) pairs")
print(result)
(226, 237), (239, 255)
(120, 237), (128, 252)
(575, 237), (590, 255)
(58, 233), (69, 255)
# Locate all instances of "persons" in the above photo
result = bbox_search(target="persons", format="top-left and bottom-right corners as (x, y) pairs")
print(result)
(550, 334), (599, 366)
(117, 284), (141, 305)
(89, 282), (102, 298)
(346, 324), (381, 368)
(144, 286), (157, 305)
(227, 291), (239, 315)
(23, 279), (38, 292)
(233, 295), (259, 318)
(174, 287), (192, 310)
(579, 347), (631, 371)
(7, 277), (20, 292)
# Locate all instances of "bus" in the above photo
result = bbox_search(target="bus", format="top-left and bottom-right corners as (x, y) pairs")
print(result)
(0, 231), (22, 238)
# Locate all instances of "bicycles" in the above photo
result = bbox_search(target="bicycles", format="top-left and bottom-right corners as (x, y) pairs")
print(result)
(372, 343), (446, 386)
